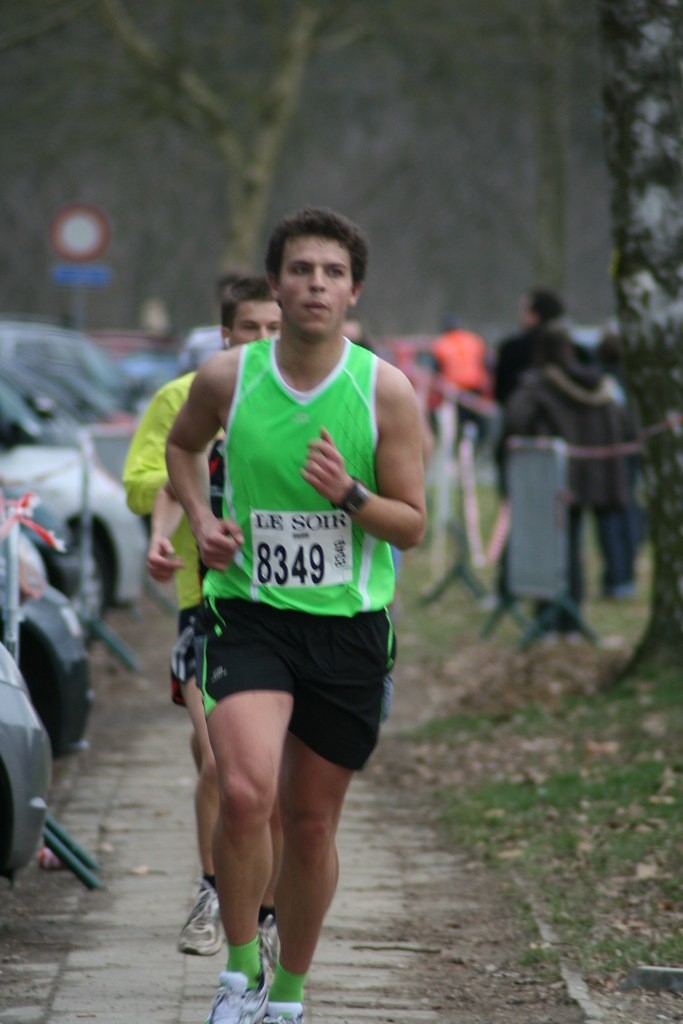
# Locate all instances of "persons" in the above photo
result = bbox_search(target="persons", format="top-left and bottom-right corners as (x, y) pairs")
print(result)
(493, 290), (636, 648)
(165, 208), (428, 1024)
(344, 319), (371, 352)
(123, 279), (286, 991)
(432, 314), (490, 450)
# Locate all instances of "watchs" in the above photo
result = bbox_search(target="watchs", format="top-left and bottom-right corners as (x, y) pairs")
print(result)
(331, 477), (368, 515)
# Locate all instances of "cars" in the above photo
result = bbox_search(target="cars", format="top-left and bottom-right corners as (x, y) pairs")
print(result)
(0, 319), (228, 884)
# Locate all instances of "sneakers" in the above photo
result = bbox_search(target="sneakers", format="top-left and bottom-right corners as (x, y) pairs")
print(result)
(256, 915), (279, 988)
(206, 953), (269, 1024)
(262, 1001), (305, 1024)
(177, 877), (225, 956)
